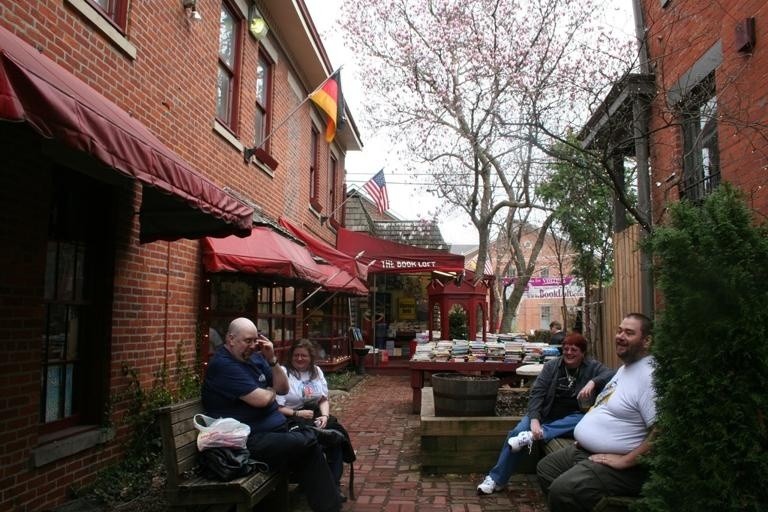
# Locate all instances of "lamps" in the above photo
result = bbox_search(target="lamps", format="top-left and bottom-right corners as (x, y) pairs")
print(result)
(184, 0), (202, 21)
(250, 16), (268, 39)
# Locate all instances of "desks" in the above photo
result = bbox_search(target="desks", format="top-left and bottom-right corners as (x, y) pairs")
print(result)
(409, 357), (532, 415)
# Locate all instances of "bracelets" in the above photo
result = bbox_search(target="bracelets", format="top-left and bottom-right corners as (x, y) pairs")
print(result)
(268, 357), (279, 367)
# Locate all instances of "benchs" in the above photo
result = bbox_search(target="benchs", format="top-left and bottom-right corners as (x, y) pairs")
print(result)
(154, 397), (291, 512)
(541, 435), (644, 512)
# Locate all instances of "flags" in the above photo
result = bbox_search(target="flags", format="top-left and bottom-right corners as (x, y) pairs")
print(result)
(309, 70), (349, 144)
(364, 171), (390, 215)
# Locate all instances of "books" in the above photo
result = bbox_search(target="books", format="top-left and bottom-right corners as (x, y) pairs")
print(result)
(414, 333), (564, 364)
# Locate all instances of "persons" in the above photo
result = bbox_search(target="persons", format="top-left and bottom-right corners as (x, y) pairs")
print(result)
(534, 312), (664, 512)
(201, 315), (340, 512)
(549, 321), (564, 345)
(208, 327), (222, 360)
(476, 332), (610, 494)
(276, 339), (354, 503)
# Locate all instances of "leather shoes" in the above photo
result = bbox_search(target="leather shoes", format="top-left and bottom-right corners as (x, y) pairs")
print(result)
(305, 424), (344, 446)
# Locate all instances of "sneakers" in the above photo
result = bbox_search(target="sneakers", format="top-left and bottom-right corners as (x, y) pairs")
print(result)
(508, 431), (533, 453)
(335, 485), (348, 502)
(476, 475), (504, 494)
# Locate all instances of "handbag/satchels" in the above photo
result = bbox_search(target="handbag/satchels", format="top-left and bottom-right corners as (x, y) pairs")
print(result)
(197, 447), (250, 482)
